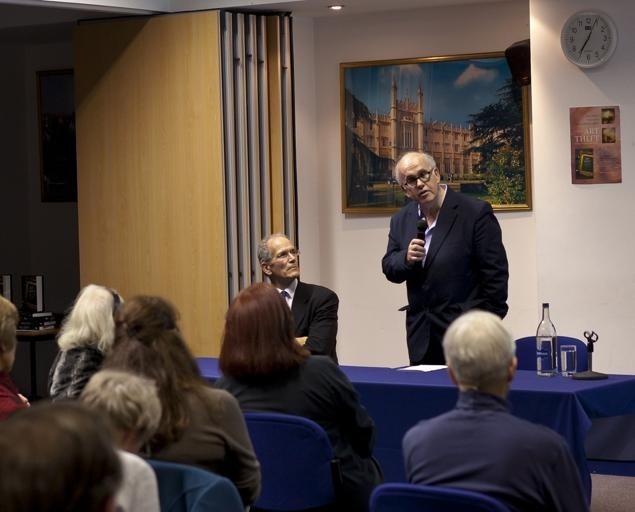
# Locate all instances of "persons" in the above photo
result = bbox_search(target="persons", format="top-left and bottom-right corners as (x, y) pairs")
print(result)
(78, 368), (164, 512)
(401, 310), (590, 512)
(46, 283), (125, 401)
(380, 150), (510, 366)
(213, 281), (385, 512)
(101, 294), (262, 509)
(0, 295), (31, 425)
(256, 233), (340, 366)
(0, 399), (124, 512)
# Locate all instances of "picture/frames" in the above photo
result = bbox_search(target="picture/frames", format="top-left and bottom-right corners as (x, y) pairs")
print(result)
(338, 50), (534, 216)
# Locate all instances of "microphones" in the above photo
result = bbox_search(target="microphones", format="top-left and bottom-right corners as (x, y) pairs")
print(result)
(416, 220), (427, 248)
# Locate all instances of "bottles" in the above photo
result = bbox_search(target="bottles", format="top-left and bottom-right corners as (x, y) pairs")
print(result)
(536, 303), (558, 377)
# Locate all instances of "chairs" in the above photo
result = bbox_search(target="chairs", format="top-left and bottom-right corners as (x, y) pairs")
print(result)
(513, 335), (592, 377)
(365, 482), (509, 512)
(234, 408), (339, 512)
(144, 461), (243, 512)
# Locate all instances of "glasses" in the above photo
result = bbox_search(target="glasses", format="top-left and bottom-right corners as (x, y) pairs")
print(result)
(404, 166), (433, 186)
(277, 249), (301, 259)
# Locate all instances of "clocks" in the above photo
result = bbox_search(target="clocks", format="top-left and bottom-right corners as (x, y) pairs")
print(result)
(559, 8), (618, 71)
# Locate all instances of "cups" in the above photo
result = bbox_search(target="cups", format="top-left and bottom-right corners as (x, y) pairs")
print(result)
(561, 345), (577, 376)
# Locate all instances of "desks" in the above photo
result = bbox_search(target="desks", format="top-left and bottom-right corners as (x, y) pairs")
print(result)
(192, 355), (635, 476)
(14, 328), (59, 400)
